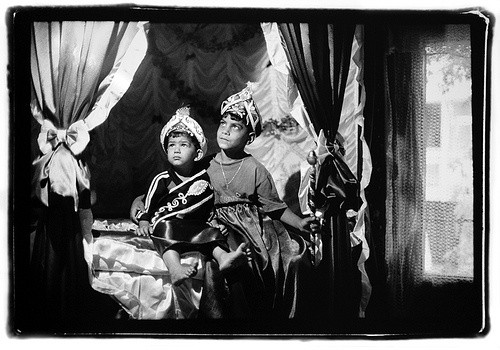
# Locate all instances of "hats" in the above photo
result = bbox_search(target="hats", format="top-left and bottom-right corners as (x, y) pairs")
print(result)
(158, 104), (208, 157)
(220, 78), (263, 137)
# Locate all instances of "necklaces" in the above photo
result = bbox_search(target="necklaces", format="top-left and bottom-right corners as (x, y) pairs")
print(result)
(217, 152), (247, 190)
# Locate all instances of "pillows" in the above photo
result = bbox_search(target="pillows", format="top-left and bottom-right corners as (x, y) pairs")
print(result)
(85, 236), (182, 276)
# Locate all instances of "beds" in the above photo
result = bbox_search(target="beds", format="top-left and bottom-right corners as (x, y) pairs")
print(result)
(29, 19), (389, 327)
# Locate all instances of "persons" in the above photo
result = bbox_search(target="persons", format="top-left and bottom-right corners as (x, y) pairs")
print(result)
(129, 87), (318, 319)
(136, 105), (252, 287)
